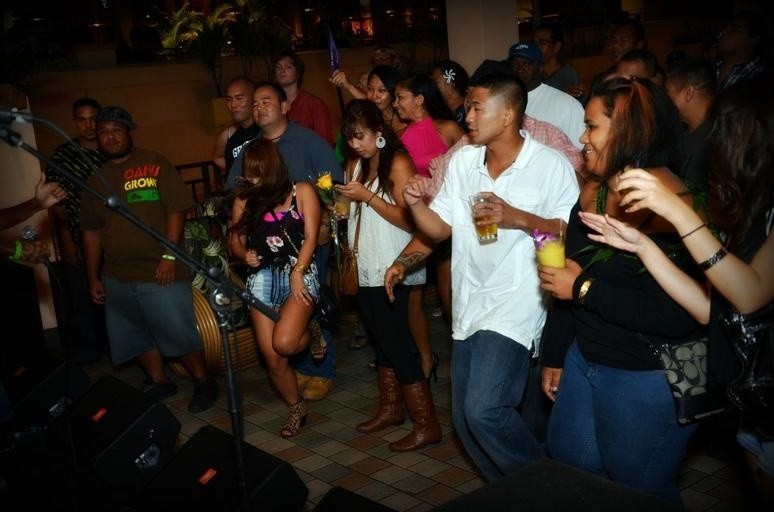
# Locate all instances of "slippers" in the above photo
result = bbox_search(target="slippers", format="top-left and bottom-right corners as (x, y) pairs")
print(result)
(355, 336), (369, 351)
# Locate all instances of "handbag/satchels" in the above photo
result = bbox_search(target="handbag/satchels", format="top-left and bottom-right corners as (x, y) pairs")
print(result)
(659, 335), (729, 425)
(707, 282), (773, 439)
(340, 242), (359, 295)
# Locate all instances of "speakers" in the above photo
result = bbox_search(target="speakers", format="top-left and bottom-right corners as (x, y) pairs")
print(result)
(47, 374), (181, 494)
(313, 486), (396, 512)
(141, 425), (308, 512)
(0, 348), (73, 444)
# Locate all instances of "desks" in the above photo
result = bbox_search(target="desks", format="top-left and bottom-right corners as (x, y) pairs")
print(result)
(418, 456), (689, 509)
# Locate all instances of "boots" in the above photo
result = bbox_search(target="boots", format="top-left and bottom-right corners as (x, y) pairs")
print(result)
(388, 378), (442, 451)
(356, 365), (405, 432)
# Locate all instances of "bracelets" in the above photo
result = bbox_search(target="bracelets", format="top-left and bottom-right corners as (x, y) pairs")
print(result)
(160, 254), (176, 261)
(9, 240), (22, 261)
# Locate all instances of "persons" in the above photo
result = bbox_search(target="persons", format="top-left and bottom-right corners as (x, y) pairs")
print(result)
(0, 166), (67, 426)
(384, 29), (770, 501)
(75, 104), (220, 415)
(38, 96), (104, 271)
(213, 46), (343, 437)
(330, 45), (470, 450)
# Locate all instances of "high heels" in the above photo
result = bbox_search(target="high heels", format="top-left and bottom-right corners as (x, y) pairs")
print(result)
(308, 318), (328, 360)
(281, 396), (307, 439)
(428, 351), (438, 389)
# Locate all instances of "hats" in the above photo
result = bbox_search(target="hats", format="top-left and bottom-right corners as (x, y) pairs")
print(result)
(509, 41), (542, 64)
(96, 105), (137, 130)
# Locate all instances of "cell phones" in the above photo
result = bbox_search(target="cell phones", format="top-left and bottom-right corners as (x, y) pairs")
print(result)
(331, 178), (345, 185)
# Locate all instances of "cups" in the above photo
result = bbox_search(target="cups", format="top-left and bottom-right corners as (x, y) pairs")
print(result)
(332, 189), (351, 220)
(467, 194), (499, 243)
(532, 229), (567, 270)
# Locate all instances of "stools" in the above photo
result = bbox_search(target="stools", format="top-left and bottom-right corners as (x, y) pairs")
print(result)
(192, 272), (340, 378)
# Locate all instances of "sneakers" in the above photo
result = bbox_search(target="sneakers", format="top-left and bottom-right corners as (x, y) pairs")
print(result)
(139, 376), (221, 413)
(368, 359), (378, 370)
(293, 369), (332, 402)
